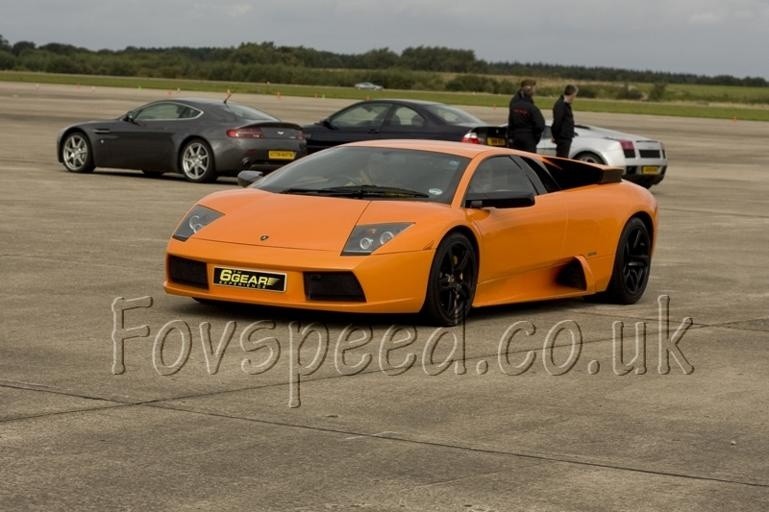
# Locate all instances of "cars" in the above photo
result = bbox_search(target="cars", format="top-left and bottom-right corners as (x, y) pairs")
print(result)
(57, 98), (307, 183)
(299, 98), (512, 155)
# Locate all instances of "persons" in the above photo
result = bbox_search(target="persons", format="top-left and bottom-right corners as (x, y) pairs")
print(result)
(509, 87), (544, 153)
(551, 84), (579, 158)
(510, 79), (536, 109)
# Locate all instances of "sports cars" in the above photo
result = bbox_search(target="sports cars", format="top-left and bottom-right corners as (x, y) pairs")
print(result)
(494, 117), (667, 188)
(163, 139), (659, 326)
